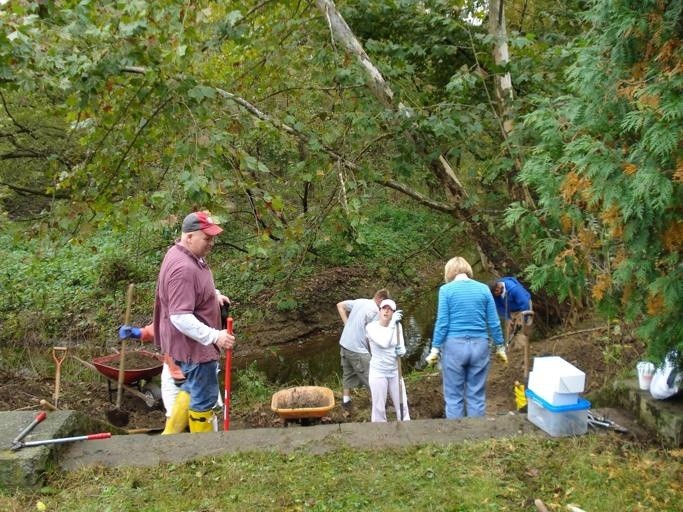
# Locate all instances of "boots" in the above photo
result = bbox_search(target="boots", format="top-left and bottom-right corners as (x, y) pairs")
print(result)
(189, 410), (215, 432)
(161, 391), (191, 434)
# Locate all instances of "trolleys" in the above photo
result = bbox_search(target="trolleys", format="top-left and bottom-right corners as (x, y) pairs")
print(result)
(72, 343), (167, 419)
(267, 381), (337, 428)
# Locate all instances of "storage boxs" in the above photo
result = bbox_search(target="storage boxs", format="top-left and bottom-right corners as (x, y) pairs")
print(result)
(524, 387), (592, 437)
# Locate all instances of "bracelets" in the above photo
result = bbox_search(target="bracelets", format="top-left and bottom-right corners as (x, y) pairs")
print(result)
(215, 289), (221, 296)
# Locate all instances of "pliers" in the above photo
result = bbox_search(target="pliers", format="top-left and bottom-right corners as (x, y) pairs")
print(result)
(2, 410), (111, 453)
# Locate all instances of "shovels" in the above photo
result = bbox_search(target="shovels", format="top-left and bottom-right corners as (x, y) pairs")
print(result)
(105, 282), (134, 428)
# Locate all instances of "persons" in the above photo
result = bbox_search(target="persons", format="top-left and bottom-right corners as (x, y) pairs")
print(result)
(155, 211), (237, 436)
(485, 276), (534, 353)
(423, 256), (508, 420)
(117, 287), (224, 432)
(334, 286), (389, 410)
(364, 298), (410, 423)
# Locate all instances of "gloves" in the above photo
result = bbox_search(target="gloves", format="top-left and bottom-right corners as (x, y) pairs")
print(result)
(119, 324), (141, 340)
(392, 310), (403, 322)
(496, 345), (507, 363)
(395, 346), (403, 357)
(425, 347), (440, 365)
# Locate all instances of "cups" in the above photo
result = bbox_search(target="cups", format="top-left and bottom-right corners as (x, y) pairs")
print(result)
(636, 361), (655, 391)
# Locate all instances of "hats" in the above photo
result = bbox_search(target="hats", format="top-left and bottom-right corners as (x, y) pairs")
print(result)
(380, 299), (396, 311)
(182, 211), (224, 236)
